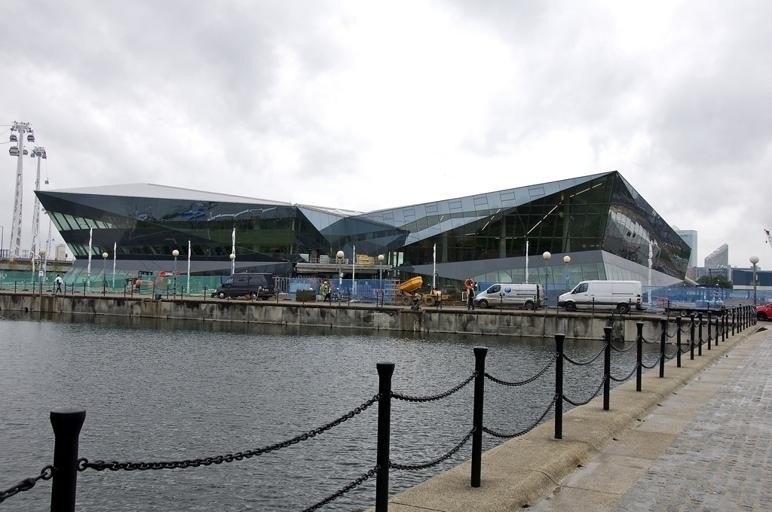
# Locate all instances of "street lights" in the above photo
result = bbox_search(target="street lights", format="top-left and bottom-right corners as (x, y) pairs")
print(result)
(337, 251), (344, 288)
(171, 249), (179, 273)
(102, 252), (109, 283)
(38, 250), (46, 271)
(709, 266), (712, 284)
(542, 251), (551, 312)
(230, 254), (236, 276)
(563, 256), (571, 293)
(378, 255), (385, 289)
(750, 255), (759, 306)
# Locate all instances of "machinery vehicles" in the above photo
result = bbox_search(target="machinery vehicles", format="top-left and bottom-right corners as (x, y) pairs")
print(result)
(394, 276), (448, 307)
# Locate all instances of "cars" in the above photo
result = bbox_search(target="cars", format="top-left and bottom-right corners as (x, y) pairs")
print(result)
(750, 304), (772, 321)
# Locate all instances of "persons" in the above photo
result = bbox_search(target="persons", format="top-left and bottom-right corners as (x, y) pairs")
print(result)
(55, 273), (63, 294)
(464, 281), (475, 309)
(323, 280), (330, 303)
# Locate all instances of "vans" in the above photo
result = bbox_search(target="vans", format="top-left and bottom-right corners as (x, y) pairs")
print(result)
(217, 273), (274, 300)
(473, 283), (544, 309)
(557, 280), (643, 314)
